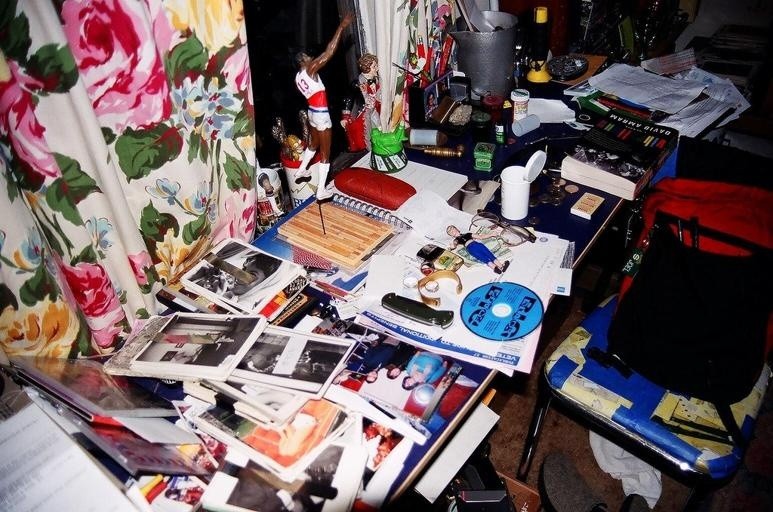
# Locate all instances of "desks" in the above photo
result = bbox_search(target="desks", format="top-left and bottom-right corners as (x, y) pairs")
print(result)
(0, 139), (677, 512)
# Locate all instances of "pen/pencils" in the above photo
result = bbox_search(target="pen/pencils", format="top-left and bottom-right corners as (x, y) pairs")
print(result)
(418, 34), (453, 85)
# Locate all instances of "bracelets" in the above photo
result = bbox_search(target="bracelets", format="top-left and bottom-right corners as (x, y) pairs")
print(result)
(418, 269), (462, 305)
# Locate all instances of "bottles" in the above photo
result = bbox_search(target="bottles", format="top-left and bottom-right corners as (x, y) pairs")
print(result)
(510, 88), (540, 137)
(513, 44), (528, 88)
(501, 150), (546, 221)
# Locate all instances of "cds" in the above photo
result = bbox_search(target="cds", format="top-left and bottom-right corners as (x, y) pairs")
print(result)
(460, 281), (545, 341)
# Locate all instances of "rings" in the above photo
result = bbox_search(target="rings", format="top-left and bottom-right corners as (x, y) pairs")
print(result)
(419, 261), (434, 276)
(402, 275), (418, 289)
(424, 280), (439, 293)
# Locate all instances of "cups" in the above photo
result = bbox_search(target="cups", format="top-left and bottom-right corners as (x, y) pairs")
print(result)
(255, 168), (288, 238)
(279, 151), (322, 211)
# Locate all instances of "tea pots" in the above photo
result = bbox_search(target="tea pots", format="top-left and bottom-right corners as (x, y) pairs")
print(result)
(447, 11), (520, 100)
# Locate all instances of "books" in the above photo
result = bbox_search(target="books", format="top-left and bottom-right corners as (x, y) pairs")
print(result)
(559, 107), (679, 202)
(250, 192), (412, 295)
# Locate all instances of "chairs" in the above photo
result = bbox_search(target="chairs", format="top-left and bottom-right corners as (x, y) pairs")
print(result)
(515, 135), (772, 511)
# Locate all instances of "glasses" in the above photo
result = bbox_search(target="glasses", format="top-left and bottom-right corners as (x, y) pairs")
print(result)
(469, 208), (536, 246)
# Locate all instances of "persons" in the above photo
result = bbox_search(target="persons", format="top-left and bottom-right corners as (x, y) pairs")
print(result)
(424, 92), (439, 121)
(293, 14), (355, 201)
(446, 224), (509, 273)
(165, 485), (202, 503)
(213, 253), (281, 297)
(332, 328), (448, 390)
(355, 53), (379, 114)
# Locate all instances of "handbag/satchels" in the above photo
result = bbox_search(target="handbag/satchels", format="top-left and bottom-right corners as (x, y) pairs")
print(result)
(608, 224), (768, 406)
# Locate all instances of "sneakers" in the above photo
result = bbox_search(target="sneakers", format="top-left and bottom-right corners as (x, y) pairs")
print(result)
(538, 452), (653, 511)
